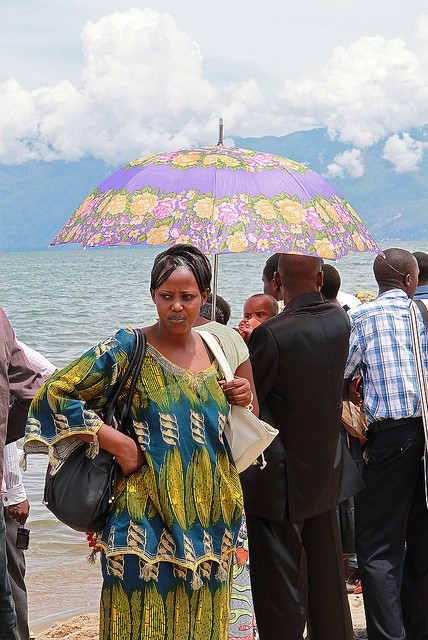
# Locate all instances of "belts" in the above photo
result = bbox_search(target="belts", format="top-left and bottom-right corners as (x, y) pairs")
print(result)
(369, 418), (421, 432)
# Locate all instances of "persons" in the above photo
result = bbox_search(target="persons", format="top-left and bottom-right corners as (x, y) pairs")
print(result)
(238, 294), (279, 342)
(202, 292), (231, 326)
(237, 254), (366, 639)
(412, 251), (428, 302)
(0, 305), (44, 638)
(344, 247), (428, 639)
(21, 244), (253, 640)
(320, 266), (354, 592)
(261, 254), (284, 314)
(1, 337), (63, 639)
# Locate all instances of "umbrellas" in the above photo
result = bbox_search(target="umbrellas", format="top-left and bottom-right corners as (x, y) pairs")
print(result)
(337, 291), (362, 310)
(45, 115), (386, 326)
(353, 289), (379, 302)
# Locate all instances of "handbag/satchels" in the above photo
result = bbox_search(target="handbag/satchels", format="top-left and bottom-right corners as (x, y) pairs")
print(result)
(342, 400), (368, 438)
(226, 404), (279, 475)
(43, 413), (130, 533)
